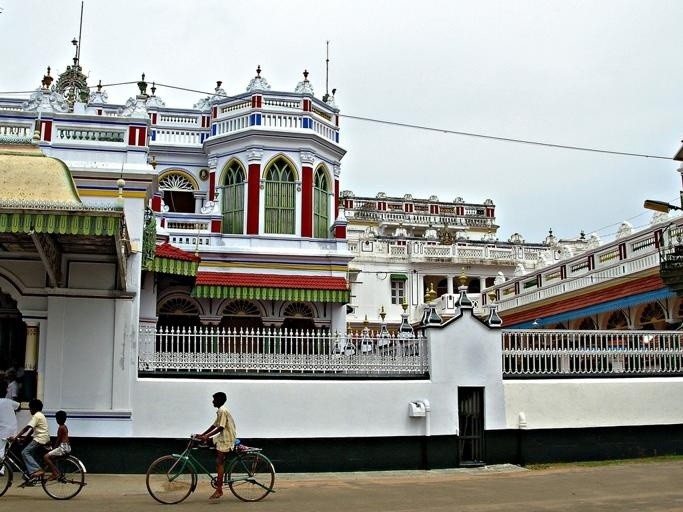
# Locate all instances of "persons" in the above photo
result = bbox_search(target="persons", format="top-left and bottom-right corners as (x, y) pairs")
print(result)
(0, 387), (22, 477)
(7, 397), (50, 487)
(42, 410), (71, 482)
(6, 375), (22, 401)
(194, 392), (236, 500)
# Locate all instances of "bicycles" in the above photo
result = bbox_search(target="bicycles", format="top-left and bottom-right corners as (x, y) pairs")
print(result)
(146, 434), (275, 505)
(0, 437), (87, 500)
